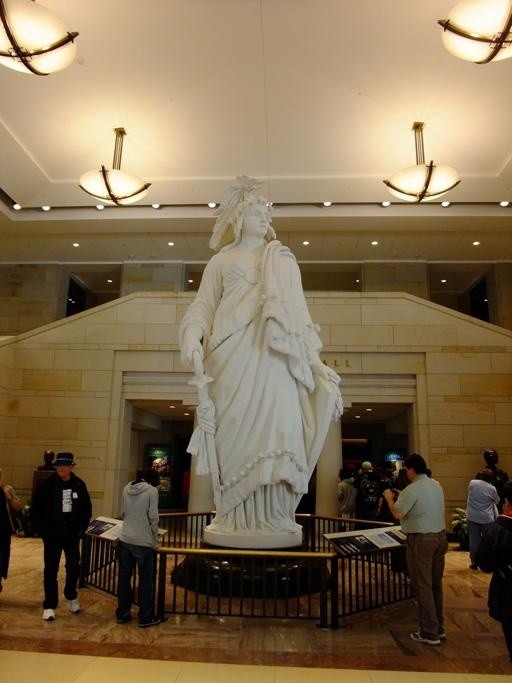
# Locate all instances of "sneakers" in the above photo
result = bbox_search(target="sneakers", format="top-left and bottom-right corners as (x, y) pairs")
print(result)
(63, 594), (80, 614)
(470, 565), (477, 570)
(117, 612), (133, 624)
(43, 609), (57, 622)
(137, 615), (161, 628)
(438, 631), (447, 638)
(410, 631), (440, 645)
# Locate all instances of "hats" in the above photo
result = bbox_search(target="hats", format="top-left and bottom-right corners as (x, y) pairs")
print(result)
(361, 461), (371, 467)
(51, 452), (77, 466)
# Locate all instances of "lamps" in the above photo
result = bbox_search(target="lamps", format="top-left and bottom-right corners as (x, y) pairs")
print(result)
(438, 1), (512, 63)
(78, 128), (153, 207)
(382, 119), (463, 207)
(0, 0), (79, 79)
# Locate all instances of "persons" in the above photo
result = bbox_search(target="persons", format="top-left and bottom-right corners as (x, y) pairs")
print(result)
(0, 468), (11, 593)
(37, 448), (58, 471)
(382, 453), (449, 646)
(353, 461), (383, 530)
(475, 448), (509, 515)
(336, 468), (358, 532)
(169, 173), (345, 549)
(3, 485), (25, 535)
(31, 452), (92, 621)
(115, 468), (163, 628)
(465, 468), (500, 570)
(474, 481), (512, 665)
